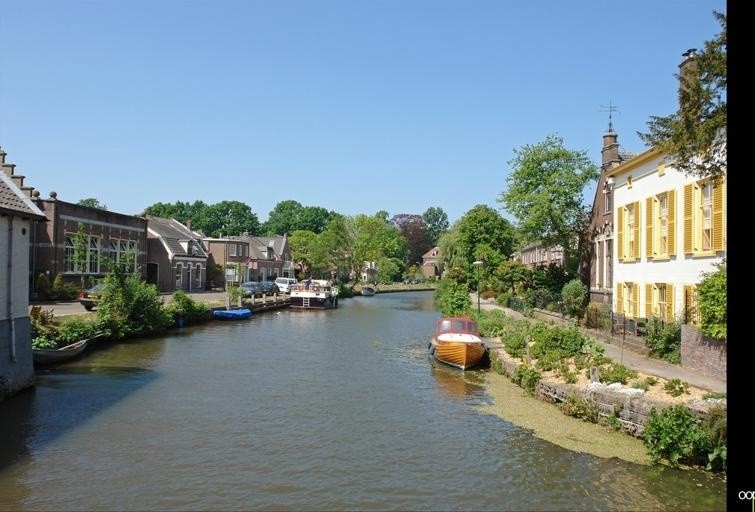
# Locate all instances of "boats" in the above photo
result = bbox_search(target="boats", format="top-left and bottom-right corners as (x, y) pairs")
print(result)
(428, 316), (489, 369)
(426, 356), (489, 404)
(288, 279), (340, 310)
(361, 286), (376, 295)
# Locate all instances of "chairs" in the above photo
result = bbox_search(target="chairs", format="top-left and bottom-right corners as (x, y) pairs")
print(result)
(612, 313), (647, 334)
(293, 282), (331, 291)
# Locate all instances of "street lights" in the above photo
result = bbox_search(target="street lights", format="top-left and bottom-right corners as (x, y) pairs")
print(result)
(473, 260), (484, 322)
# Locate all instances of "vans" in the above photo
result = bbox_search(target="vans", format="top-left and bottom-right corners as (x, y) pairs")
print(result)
(274, 277), (299, 294)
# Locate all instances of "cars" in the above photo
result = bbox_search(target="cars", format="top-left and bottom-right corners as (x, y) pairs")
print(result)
(237, 281), (264, 299)
(404, 278), (422, 284)
(79, 283), (105, 311)
(259, 282), (279, 296)
